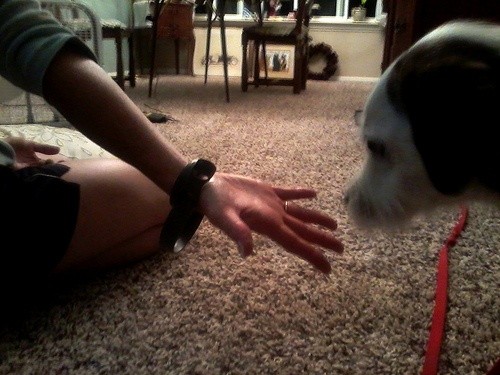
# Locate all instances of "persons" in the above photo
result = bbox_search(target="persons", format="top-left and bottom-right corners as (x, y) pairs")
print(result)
(0, 1), (344, 331)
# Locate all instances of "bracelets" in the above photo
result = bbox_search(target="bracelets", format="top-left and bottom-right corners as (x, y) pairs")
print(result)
(160, 158), (216, 255)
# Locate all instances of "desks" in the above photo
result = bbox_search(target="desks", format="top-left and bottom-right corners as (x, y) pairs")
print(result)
(66, 19), (136, 91)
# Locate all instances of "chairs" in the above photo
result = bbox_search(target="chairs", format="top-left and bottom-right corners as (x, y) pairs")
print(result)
(241, 0), (319, 94)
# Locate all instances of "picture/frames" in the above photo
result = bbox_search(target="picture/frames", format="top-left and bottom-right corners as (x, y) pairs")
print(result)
(192, 21), (296, 78)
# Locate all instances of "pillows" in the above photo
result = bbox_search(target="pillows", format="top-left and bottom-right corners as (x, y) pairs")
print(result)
(0, 123), (119, 164)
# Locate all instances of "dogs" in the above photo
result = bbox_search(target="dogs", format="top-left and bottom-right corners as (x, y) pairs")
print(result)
(342, 18), (500, 233)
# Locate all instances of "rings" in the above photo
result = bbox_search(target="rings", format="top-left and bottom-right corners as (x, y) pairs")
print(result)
(285, 200), (288, 210)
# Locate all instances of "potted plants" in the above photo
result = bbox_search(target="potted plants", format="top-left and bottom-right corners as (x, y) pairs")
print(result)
(351, 0), (367, 21)
